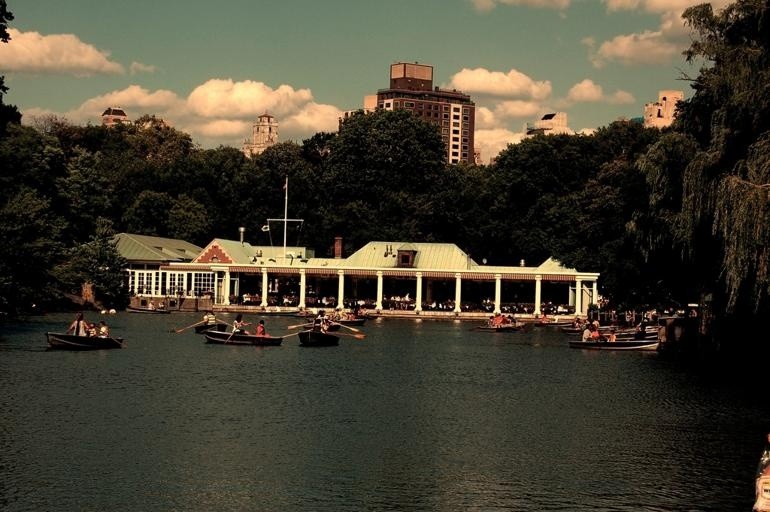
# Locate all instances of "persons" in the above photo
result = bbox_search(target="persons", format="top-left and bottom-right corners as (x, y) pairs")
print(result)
(203, 310), (215, 325)
(483, 298), (568, 325)
(384, 294), (453, 311)
(149, 301), (164, 310)
(322, 295), (375, 310)
(237, 292), (296, 306)
(256, 320), (265, 335)
(582, 308), (673, 341)
(233, 314), (251, 334)
(71, 312), (109, 338)
(311, 305), (368, 335)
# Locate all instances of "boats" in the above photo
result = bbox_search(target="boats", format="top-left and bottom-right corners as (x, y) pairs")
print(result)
(194, 324), (228, 335)
(298, 331), (340, 346)
(304, 324), (340, 332)
(47, 332), (124, 350)
(478, 323), (534, 332)
(536, 323), (659, 351)
(331, 318), (365, 326)
(204, 329), (283, 346)
(127, 305), (170, 315)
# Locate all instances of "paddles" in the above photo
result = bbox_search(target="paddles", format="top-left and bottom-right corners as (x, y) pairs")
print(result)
(326, 332), (364, 339)
(176, 320), (208, 333)
(288, 323), (312, 329)
(336, 322), (359, 332)
(95, 327), (129, 349)
